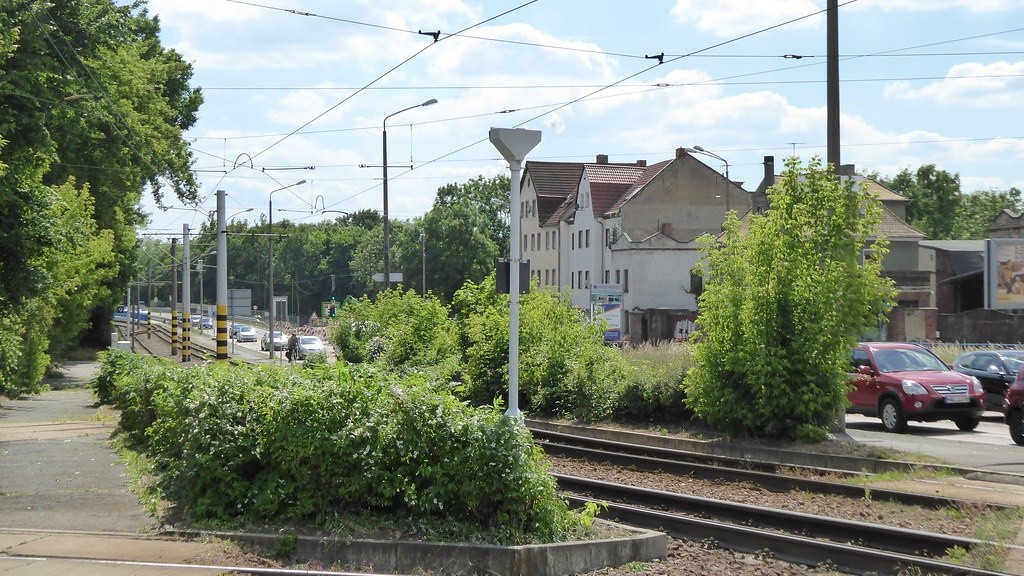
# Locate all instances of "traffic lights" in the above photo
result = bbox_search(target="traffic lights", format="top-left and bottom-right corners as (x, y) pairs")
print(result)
(328, 307), (335, 317)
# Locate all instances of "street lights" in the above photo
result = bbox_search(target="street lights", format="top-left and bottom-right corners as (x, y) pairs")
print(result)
(380, 97), (438, 295)
(265, 180), (309, 362)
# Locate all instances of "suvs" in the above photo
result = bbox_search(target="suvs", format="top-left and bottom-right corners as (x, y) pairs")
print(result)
(843, 338), (984, 436)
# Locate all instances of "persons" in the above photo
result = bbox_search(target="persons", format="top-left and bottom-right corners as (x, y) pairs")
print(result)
(285, 333), (299, 363)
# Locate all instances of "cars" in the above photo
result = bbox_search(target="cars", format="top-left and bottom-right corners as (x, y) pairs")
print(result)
(177, 311), (259, 343)
(1001, 362), (1024, 447)
(296, 335), (327, 359)
(261, 331), (289, 352)
(950, 349), (1024, 416)
